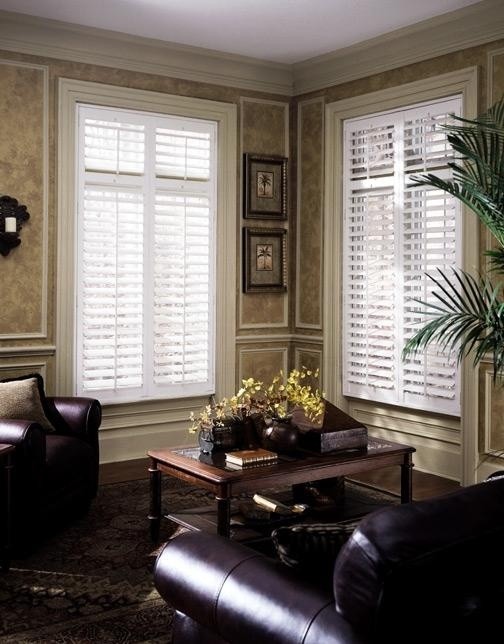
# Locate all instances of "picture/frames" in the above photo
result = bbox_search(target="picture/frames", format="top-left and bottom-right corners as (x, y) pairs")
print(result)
(244, 226), (288, 295)
(243, 152), (287, 221)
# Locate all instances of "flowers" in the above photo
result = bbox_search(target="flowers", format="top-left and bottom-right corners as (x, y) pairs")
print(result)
(188, 366), (328, 434)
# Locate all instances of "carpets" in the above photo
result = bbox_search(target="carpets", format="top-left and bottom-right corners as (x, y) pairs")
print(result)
(0, 476), (418, 644)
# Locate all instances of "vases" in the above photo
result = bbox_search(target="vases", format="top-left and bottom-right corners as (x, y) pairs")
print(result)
(199, 419), (300, 456)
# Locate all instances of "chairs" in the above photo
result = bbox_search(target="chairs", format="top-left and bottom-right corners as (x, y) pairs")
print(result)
(0, 398), (102, 531)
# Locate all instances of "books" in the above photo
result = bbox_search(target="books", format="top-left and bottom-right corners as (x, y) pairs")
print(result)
(224, 447), (278, 466)
(252, 493), (291, 512)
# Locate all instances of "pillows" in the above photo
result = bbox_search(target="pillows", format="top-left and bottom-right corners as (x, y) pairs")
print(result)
(0, 374), (58, 434)
(270, 521), (360, 590)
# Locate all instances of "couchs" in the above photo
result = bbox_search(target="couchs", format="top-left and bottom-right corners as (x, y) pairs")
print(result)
(154, 476), (504, 644)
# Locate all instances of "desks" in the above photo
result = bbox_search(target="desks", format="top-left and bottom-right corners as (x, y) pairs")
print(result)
(0, 444), (16, 511)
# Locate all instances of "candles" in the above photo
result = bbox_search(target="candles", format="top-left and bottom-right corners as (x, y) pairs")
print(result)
(5, 217), (17, 232)
